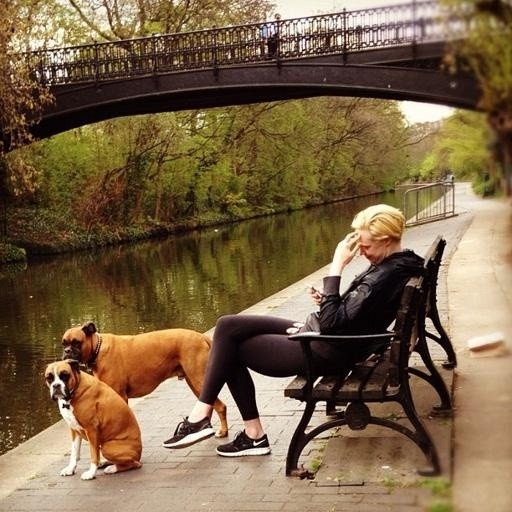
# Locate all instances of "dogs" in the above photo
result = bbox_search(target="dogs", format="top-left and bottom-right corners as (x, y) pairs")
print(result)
(37, 358), (143, 482)
(60, 320), (229, 439)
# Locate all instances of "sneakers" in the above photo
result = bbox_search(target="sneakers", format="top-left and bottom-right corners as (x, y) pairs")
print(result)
(163, 416), (216, 450)
(215, 429), (271, 456)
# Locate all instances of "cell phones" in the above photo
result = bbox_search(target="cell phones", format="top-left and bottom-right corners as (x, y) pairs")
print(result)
(307, 282), (321, 297)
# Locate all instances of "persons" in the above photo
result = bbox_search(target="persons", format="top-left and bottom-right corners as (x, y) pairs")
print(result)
(258, 12), (282, 59)
(161, 203), (425, 458)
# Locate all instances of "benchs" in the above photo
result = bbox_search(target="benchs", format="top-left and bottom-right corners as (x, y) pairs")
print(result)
(282, 234), (458, 476)
